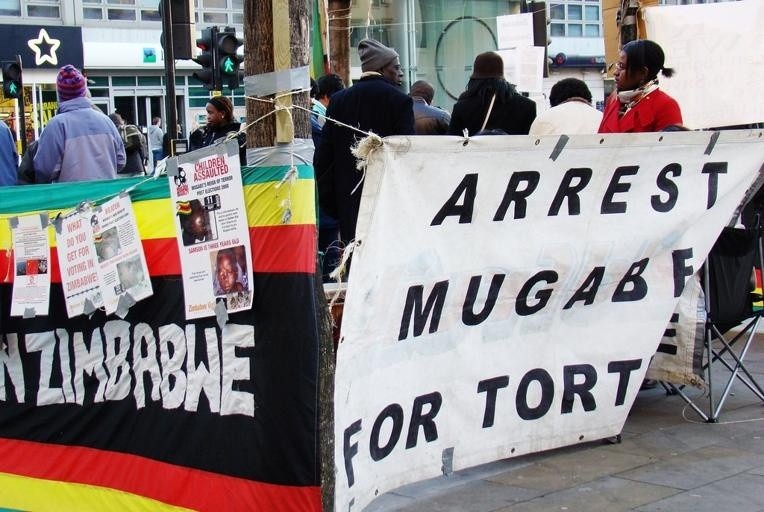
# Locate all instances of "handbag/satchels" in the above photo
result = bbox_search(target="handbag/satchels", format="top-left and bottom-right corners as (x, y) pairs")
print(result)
(117, 124), (144, 156)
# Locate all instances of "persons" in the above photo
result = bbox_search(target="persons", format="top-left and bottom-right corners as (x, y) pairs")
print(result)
(305, 38), (684, 283)
(1, 64), (248, 186)
(215, 251), (244, 293)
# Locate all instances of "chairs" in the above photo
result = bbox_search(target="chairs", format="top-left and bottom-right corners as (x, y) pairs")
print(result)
(657, 225), (763, 423)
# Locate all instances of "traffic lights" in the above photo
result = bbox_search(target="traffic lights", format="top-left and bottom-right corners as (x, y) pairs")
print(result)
(1, 61), (23, 98)
(192, 24), (244, 90)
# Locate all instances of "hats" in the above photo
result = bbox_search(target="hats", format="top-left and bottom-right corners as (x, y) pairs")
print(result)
(469, 51), (504, 81)
(357, 37), (399, 74)
(55, 64), (88, 102)
